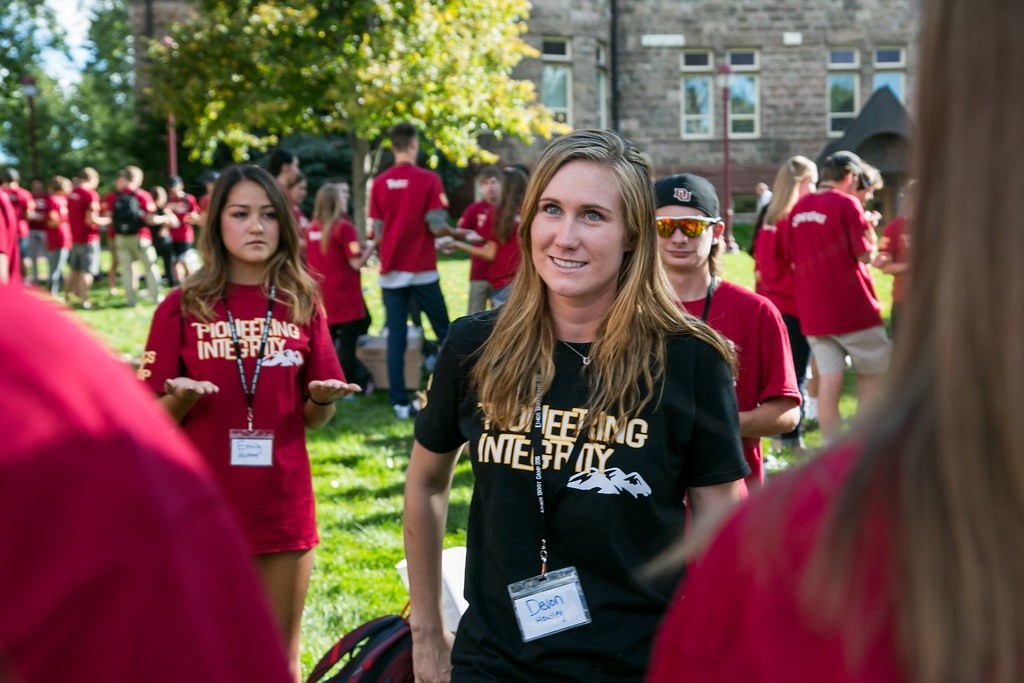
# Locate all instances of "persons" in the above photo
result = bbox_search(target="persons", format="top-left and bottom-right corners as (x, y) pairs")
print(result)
(402, 128), (751, 683)
(653, 171), (803, 490)
(746, 151), (920, 453)
(0, 282), (297, 683)
(268, 146), (372, 400)
(140, 164), (363, 683)
(369, 122), (452, 419)
(1, 165), (220, 310)
(646, 0), (1024, 683)
(457, 162), (527, 315)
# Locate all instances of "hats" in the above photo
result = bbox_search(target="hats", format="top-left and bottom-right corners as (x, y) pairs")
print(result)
(823, 150), (864, 173)
(202, 171), (222, 184)
(166, 174), (183, 188)
(653, 172), (721, 219)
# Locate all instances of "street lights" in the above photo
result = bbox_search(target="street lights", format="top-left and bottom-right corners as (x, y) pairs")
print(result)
(713, 61), (739, 254)
(19, 74), (40, 178)
(160, 33), (180, 179)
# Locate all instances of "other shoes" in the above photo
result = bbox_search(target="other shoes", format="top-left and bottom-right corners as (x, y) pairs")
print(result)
(393, 403), (412, 418)
(765, 452), (787, 470)
(423, 354), (437, 371)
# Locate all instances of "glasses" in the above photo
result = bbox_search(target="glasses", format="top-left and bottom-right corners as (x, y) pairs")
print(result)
(654, 215), (717, 239)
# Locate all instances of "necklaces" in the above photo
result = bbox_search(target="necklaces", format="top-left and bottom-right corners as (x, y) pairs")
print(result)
(554, 336), (593, 366)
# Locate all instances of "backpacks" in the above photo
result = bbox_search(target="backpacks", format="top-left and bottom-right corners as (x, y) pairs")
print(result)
(108, 187), (147, 236)
(304, 599), (414, 683)
